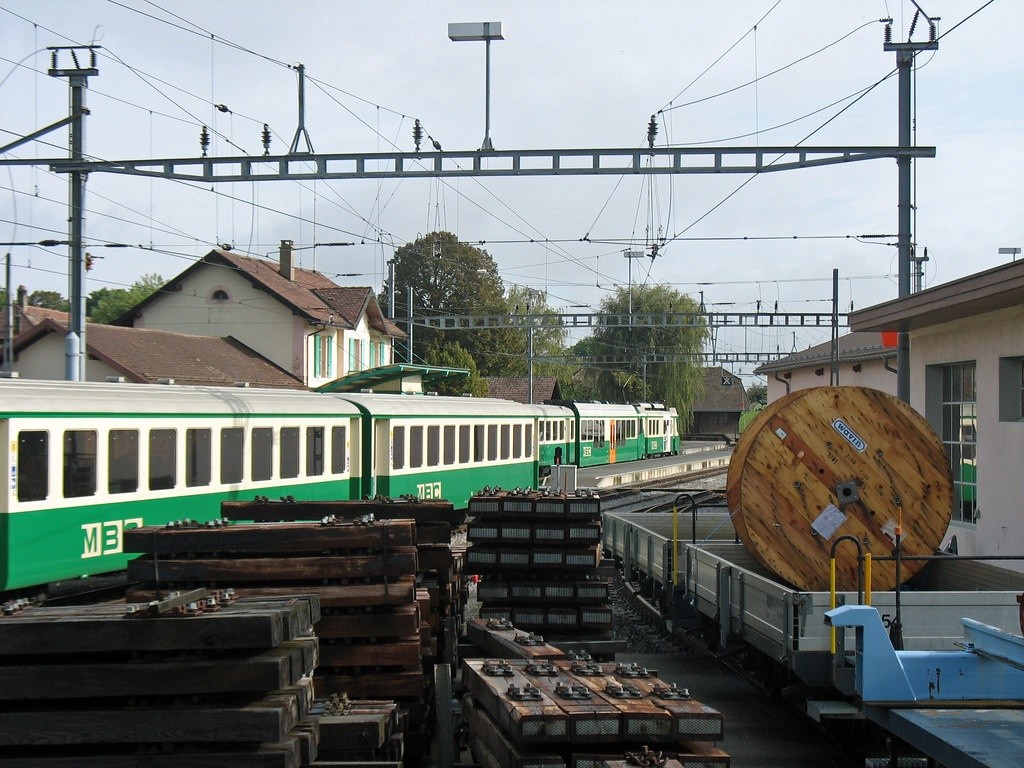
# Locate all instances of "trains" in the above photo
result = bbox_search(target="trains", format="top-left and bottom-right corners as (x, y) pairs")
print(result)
(0, 373), (680, 600)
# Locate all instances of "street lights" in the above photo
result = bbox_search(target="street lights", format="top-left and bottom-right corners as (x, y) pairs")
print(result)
(622, 251), (646, 328)
